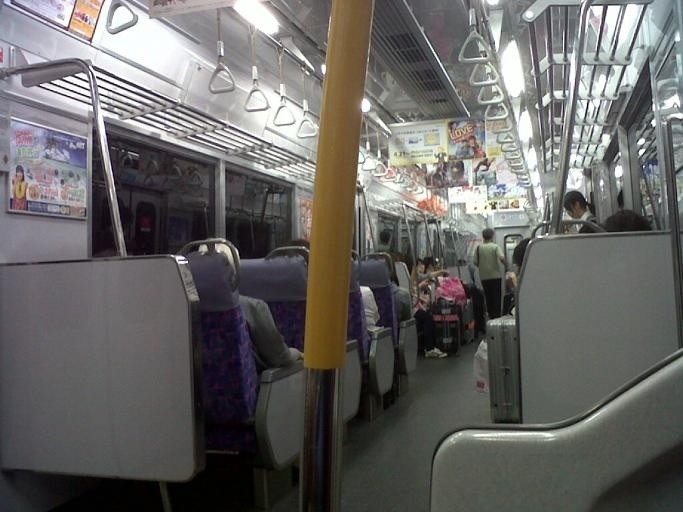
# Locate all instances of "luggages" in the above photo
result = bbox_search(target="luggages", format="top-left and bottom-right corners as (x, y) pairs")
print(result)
(431, 275), (462, 357)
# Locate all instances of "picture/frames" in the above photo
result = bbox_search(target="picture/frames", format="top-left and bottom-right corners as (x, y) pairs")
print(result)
(6, 115), (89, 220)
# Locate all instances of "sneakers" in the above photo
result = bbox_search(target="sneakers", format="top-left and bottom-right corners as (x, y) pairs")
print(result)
(425, 347), (448, 359)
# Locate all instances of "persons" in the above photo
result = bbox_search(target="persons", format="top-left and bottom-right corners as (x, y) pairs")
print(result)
(11, 165), (29, 210)
(564, 190), (595, 231)
(603, 209), (655, 233)
(472, 237), (532, 410)
(357, 286), (381, 330)
(371, 226), (450, 359)
(200, 238), (305, 376)
(472, 225), (509, 321)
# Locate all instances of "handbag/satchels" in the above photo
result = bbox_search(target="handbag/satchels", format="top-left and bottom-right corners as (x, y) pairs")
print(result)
(432, 277), (467, 314)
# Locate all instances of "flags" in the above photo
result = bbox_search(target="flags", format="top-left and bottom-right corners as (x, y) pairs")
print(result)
(387, 118), (532, 216)
